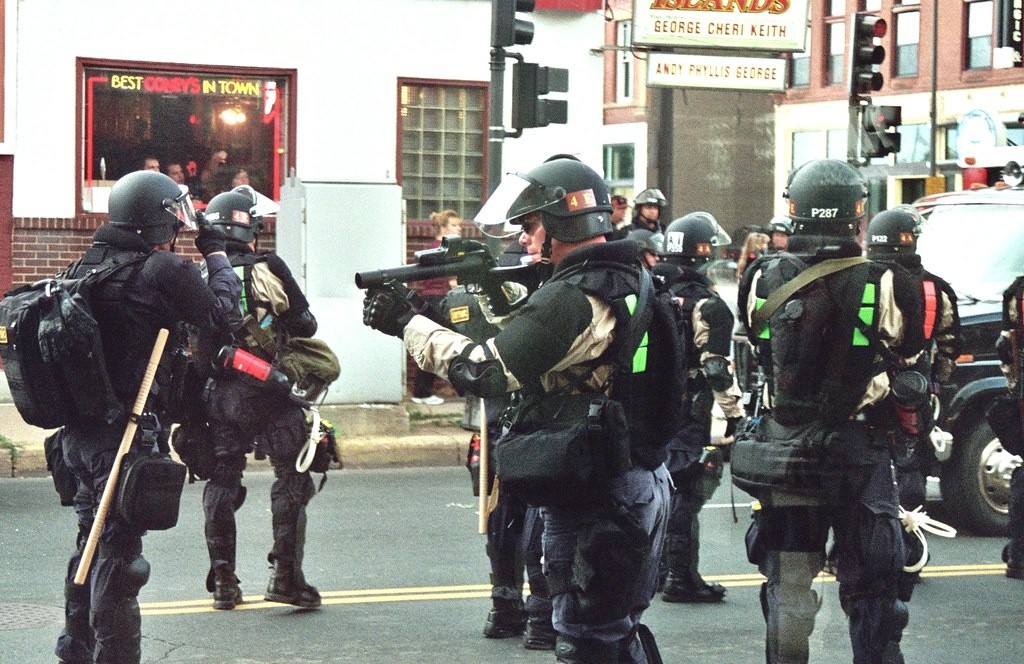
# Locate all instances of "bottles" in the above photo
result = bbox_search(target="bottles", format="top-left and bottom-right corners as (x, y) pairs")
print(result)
(214, 346), (290, 397)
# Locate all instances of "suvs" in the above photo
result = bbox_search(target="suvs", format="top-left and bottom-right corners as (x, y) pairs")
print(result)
(910, 188), (1023, 537)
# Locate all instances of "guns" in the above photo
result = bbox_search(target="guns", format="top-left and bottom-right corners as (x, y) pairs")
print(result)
(353, 233), (539, 317)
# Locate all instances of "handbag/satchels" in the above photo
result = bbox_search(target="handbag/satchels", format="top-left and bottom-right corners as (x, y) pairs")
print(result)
(279, 337), (340, 389)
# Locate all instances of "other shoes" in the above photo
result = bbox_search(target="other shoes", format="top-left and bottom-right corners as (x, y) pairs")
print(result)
(1006, 544), (1024, 578)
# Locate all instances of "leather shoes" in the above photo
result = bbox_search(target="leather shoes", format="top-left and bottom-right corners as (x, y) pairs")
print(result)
(523, 613), (556, 648)
(265, 560), (322, 607)
(661, 574), (725, 603)
(485, 598), (529, 637)
(212, 574), (243, 609)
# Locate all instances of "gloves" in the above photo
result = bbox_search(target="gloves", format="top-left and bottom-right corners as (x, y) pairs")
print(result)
(363, 289), (419, 338)
(724, 417), (744, 439)
(194, 209), (227, 257)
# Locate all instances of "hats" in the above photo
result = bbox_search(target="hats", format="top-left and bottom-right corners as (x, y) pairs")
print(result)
(610, 194), (628, 209)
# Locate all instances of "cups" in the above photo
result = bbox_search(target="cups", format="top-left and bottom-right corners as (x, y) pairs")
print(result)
(891, 371), (932, 438)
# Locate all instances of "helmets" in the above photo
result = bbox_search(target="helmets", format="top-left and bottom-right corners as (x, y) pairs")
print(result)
(768, 215), (794, 236)
(108, 170), (186, 244)
(787, 160), (868, 222)
(866, 207), (924, 247)
(635, 192), (662, 207)
(204, 192), (264, 242)
(661, 216), (718, 257)
(506, 153), (614, 244)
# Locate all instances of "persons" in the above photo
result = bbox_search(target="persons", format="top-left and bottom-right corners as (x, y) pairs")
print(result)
(1000, 275), (1024, 579)
(866, 204), (961, 584)
(134, 146), (264, 203)
(411, 210), (461, 404)
(484, 155), (747, 651)
(193, 186), (322, 608)
(45, 171), (243, 664)
(362, 158), (681, 664)
(736, 161), (923, 664)
(738, 223), (790, 276)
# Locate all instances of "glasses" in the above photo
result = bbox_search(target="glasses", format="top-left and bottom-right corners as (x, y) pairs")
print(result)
(518, 215), (541, 236)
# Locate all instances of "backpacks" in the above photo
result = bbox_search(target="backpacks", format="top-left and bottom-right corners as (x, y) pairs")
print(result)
(0, 248), (148, 429)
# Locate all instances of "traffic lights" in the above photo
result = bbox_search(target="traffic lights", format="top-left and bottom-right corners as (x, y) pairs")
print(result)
(492, 1), (534, 48)
(851, 13), (886, 98)
(861, 104), (901, 157)
(512, 63), (549, 129)
